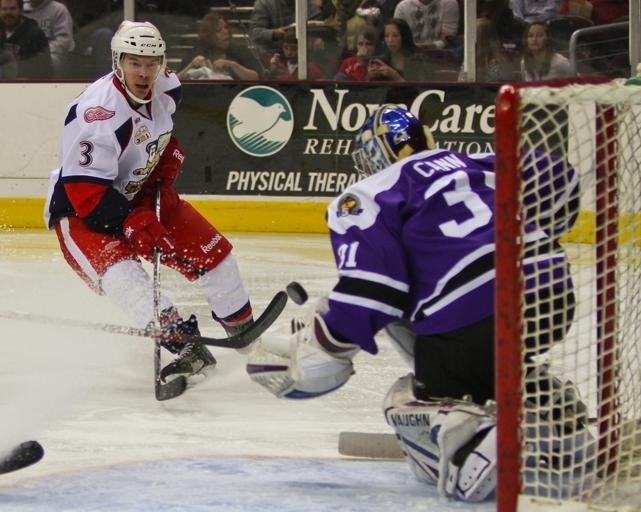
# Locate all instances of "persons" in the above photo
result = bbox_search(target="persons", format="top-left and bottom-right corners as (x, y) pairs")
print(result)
(179, 13), (264, 82)
(250, 1), (593, 82)
(1, 1), (210, 83)
(42, 17), (260, 400)
(246, 106), (601, 503)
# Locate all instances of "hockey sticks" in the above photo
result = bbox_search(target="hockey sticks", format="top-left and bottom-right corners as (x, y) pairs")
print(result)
(152, 178), (187, 400)
(1, 291), (287, 347)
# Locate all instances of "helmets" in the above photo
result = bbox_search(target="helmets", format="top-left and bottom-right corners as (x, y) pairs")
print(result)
(111, 17), (167, 81)
(355, 103), (428, 168)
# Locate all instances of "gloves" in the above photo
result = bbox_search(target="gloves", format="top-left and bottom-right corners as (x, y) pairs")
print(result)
(119, 205), (176, 263)
(140, 185), (180, 216)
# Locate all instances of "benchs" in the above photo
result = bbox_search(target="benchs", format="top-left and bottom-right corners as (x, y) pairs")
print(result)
(167, 0), (255, 73)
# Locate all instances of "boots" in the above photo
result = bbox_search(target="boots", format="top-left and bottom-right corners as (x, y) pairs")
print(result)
(159, 314), (218, 384)
